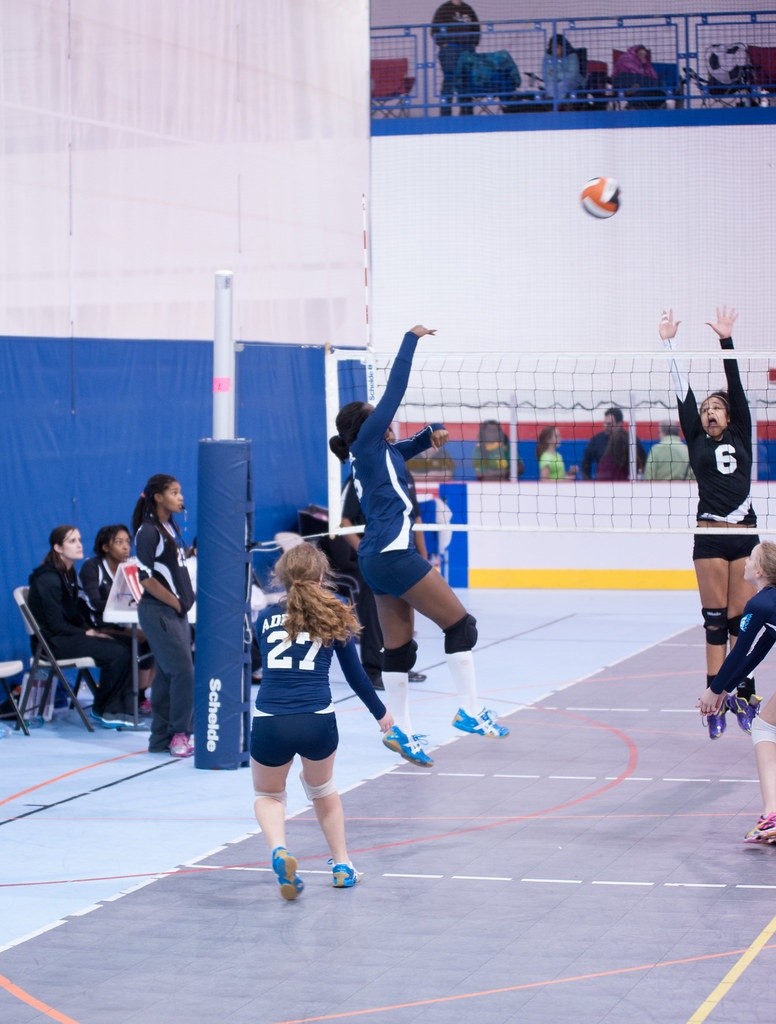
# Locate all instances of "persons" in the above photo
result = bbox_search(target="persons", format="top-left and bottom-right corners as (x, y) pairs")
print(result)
(645, 416), (696, 480)
(328, 324), (508, 769)
(694, 541), (776, 844)
(539, 32), (590, 110)
(705, 42), (771, 106)
(130, 473), (195, 757)
(430, 0), (481, 116)
(251, 541), (394, 900)
(476, 416), (523, 480)
(535, 424), (579, 482)
(583, 407), (647, 481)
(26, 525), (144, 728)
(660, 304), (764, 740)
(341, 425), (426, 688)
(611, 45), (667, 109)
(79, 524), (145, 712)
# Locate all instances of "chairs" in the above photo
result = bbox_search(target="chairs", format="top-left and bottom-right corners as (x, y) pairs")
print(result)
(586, 60), (616, 110)
(453, 51), (522, 116)
(12, 586), (98, 733)
(682, 67), (745, 108)
(744, 44), (776, 104)
(651, 62), (677, 86)
(612, 48), (667, 110)
(0, 660), (30, 736)
(370, 57), (417, 119)
(524, 46), (587, 111)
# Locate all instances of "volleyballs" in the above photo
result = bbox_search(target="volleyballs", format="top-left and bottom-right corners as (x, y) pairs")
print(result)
(580, 176), (624, 221)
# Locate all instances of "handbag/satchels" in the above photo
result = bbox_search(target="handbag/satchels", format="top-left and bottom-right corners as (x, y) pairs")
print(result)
(19, 666), (58, 722)
(704, 40), (749, 93)
(454, 50), (521, 95)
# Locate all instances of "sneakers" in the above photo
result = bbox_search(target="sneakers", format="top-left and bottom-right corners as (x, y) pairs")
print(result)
(452, 704), (510, 738)
(328, 858), (362, 887)
(700, 693), (730, 741)
(383, 723), (435, 768)
(272, 847), (305, 900)
(725, 693), (762, 734)
(744, 813), (775, 845)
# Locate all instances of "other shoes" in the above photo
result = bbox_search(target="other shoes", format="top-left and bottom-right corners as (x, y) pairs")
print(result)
(90, 706), (103, 720)
(139, 700), (151, 717)
(406, 671), (426, 682)
(371, 676), (384, 691)
(102, 710), (145, 729)
(167, 731), (195, 757)
